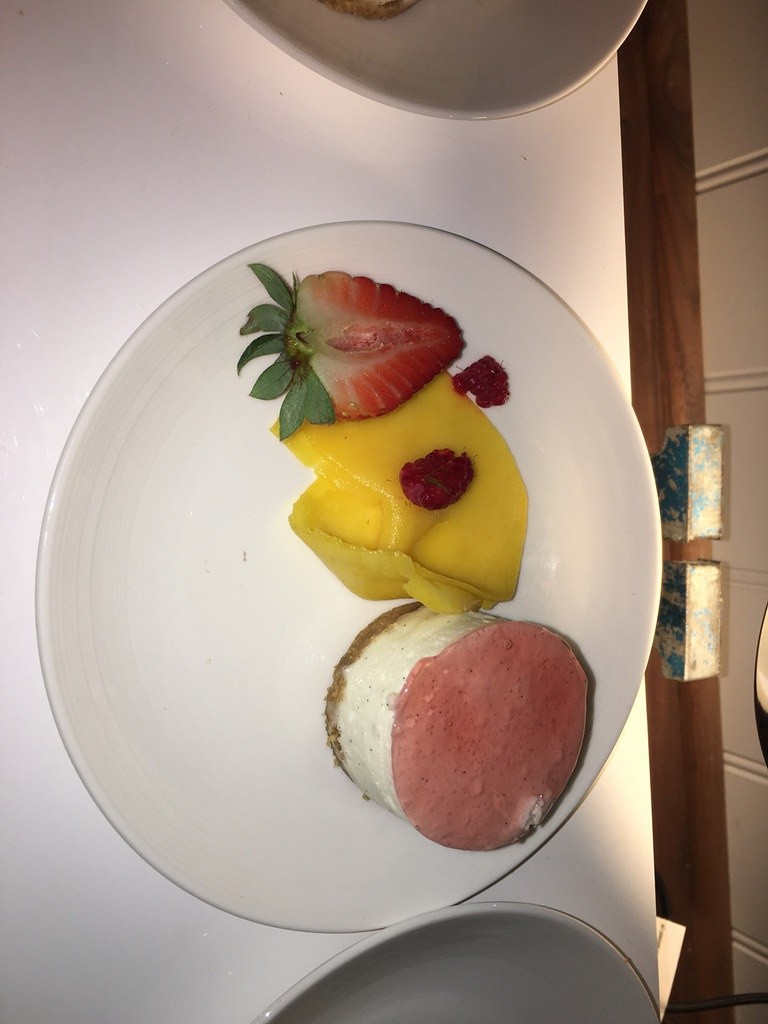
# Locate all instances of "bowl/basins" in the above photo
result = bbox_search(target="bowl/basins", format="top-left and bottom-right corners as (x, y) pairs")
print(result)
(224, 0), (649, 121)
(253, 902), (664, 1024)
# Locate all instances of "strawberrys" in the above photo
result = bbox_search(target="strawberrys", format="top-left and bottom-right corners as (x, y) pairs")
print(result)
(296, 271), (466, 421)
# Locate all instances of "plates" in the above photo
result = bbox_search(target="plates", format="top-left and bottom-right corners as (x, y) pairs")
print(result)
(37, 220), (663, 934)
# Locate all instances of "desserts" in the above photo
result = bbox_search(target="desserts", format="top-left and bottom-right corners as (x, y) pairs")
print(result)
(321, 600), (591, 852)
